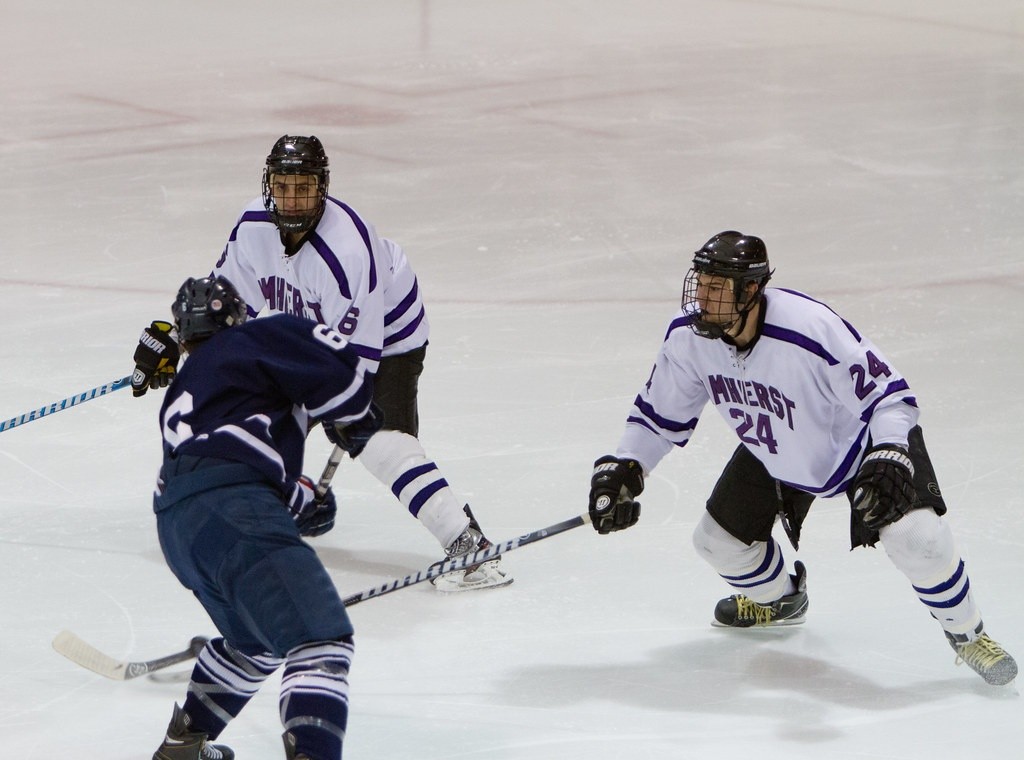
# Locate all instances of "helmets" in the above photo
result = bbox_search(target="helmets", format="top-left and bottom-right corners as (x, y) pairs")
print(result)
(682, 231), (776, 340)
(262, 134), (330, 233)
(171, 275), (246, 354)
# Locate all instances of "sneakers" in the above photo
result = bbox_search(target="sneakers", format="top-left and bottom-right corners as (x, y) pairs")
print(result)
(151, 700), (235, 760)
(711, 560), (808, 628)
(428, 504), (514, 593)
(930, 610), (1022, 697)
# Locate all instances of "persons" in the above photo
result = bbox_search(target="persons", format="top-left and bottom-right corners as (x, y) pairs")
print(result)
(589, 231), (1016, 686)
(152, 272), (383, 760)
(130, 134), (514, 592)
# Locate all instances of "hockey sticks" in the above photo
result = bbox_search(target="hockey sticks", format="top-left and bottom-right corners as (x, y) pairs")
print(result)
(317, 439), (347, 495)
(51, 495), (630, 682)
(0, 373), (137, 432)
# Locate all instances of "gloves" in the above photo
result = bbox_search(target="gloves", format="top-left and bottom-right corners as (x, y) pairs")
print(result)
(588, 455), (645, 535)
(320, 402), (385, 460)
(131, 320), (181, 397)
(295, 487), (335, 535)
(850, 443), (916, 529)
